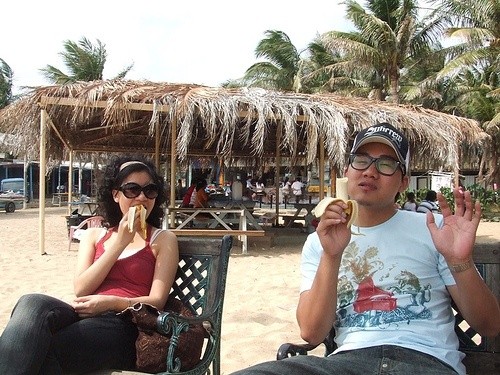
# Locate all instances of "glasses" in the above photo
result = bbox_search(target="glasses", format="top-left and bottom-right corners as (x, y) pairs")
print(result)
(111, 183), (160, 200)
(347, 152), (404, 177)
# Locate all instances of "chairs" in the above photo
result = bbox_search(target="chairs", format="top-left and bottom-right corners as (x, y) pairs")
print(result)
(276, 235), (500, 375)
(116, 233), (232, 375)
(68, 216), (104, 250)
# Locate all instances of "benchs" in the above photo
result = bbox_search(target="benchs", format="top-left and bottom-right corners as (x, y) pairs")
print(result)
(159, 215), (307, 253)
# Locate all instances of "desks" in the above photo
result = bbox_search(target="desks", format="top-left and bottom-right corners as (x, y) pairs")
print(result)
(167, 208), (246, 232)
(288, 202), (317, 213)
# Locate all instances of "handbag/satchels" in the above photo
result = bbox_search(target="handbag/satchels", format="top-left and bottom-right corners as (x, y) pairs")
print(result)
(116, 298), (207, 373)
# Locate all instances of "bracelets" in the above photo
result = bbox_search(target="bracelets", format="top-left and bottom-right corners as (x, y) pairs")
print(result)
(448, 258), (473, 272)
(127, 298), (131, 308)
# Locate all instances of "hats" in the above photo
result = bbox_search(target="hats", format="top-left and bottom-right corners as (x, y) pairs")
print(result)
(428, 191), (436, 200)
(348, 122), (410, 175)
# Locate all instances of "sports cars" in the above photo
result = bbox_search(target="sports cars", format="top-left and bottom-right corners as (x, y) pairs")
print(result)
(0, 191), (24, 213)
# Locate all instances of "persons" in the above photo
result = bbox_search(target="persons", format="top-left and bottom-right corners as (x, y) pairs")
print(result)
(256, 179), (266, 196)
(282, 176), (291, 198)
(401, 192), (419, 212)
(87, 181), (91, 196)
(179, 177), (211, 228)
(0, 155), (179, 375)
(178, 179), (182, 199)
(247, 176), (255, 193)
(228, 123), (500, 375)
(416, 190), (439, 214)
(291, 177), (304, 203)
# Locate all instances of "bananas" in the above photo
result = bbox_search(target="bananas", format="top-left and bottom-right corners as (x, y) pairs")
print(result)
(310, 177), (358, 229)
(128, 204), (147, 239)
